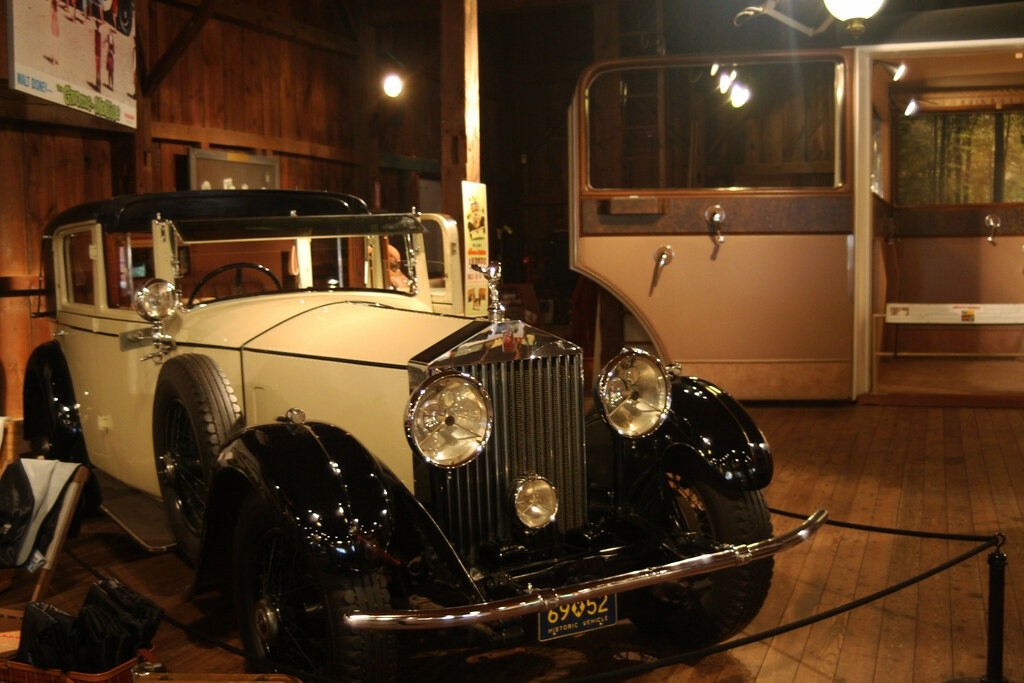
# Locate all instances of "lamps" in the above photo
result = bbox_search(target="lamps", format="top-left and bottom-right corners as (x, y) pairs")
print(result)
(732, 0), (885, 41)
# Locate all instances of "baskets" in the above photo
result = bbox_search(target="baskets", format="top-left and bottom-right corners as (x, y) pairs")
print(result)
(0, 641), (156, 683)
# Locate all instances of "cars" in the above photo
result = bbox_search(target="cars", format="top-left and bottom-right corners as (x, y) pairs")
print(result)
(22, 191), (830, 678)
